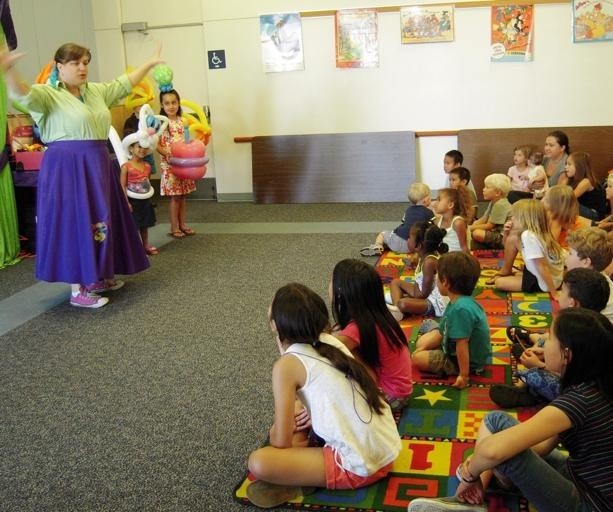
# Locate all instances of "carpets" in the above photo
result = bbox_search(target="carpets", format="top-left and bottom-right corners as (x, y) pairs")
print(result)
(232, 246), (569, 512)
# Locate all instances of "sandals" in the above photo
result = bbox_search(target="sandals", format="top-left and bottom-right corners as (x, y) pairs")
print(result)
(506, 324), (544, 345)
(145, 246), (160, 255)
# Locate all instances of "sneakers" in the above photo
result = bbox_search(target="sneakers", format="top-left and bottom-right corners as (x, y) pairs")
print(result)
(421, 320), (439, 330)
(71, 285), (109, 308)
(407, 496), (489, 512)
(246, 480), (315, 508)
(360, 242), (385, 258)
(385, 303), (403, 321)
(385, 293), (394, 302)
(88, 277), (124, 294)
(486, 479), (520, 494)
(488, 383), (543, 409)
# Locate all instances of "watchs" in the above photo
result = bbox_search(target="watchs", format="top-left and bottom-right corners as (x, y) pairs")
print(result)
(456, 464), (481, 487)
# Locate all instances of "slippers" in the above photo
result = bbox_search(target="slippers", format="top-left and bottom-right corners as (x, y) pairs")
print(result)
(182, 226), (195, 236)
(485, 273), (515, 287)
(520, 263), (525, 272)
(168, 231), (185, 239)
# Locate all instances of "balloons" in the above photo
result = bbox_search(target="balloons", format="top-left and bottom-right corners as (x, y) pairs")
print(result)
(123, 62), (155, 110)
(169, 123), (209, 181)
(153, 64), (173, 92)
(179, 100), (211, 146)
(108, 104), (169, 200)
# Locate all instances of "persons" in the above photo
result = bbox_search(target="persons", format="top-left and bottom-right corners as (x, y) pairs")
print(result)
(412, 251), (491, 389)
(507, 146), (532, 192)
(1, 39), (167, 309)
(507, 131), (575, 205)
(327, 258), (413, 411)
(386, 220), (451, 322)
(488, 267), (613, 409)
(157, 89), (196, 239)
(469, 173), (514, 245)
(506, 225), (613, 367)
(527, 151), (549, 199)
(565, 151), (612, 222)
(359, 183), (437, 257)
(543, 185), (580, 252)
(123, 94), (157, 207)
(598, 169), (613, 230)
(444, 150), (478, 217)
(246, 282), (402, 509)
(486, 199), (564, 303)
(409, 184), (473, 271)
(407, 307), (613, 512)
(437, 167), (479, 226)
(120, 140), (159, 256)
